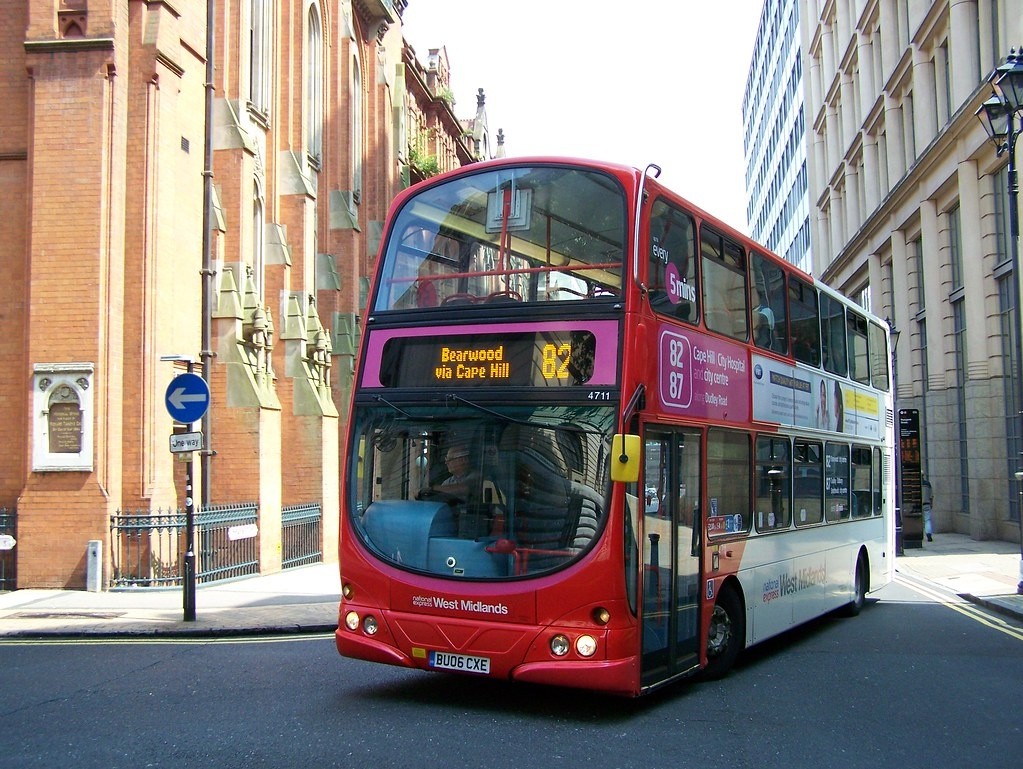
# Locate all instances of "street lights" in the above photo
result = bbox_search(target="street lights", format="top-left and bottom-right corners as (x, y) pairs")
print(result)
(974, 45), (1022, 596)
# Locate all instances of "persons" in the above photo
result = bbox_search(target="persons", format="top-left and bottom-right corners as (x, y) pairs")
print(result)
(815, 379), (830, 429)
(422, 441), (483, 510)
(833, 380), (844, 432)
(755, 306), (783, 353)
(920, 469), (934, 542)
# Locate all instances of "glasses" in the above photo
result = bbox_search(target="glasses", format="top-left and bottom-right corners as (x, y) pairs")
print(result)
(445, 455), (468, 462)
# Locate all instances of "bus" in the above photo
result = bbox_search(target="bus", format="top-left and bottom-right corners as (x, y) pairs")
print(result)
(336, 155), (897, 700)
